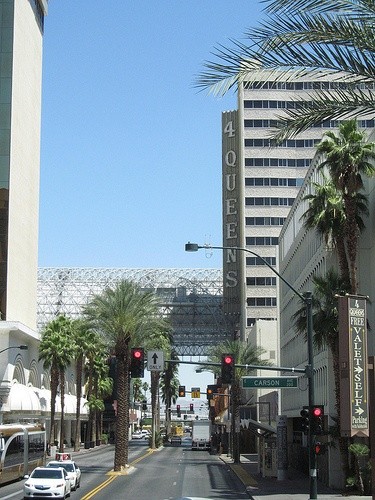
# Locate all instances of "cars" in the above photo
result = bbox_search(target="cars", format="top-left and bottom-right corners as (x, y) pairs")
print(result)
(185, 429), (192, 433)
(46, 460), (81, 491)
(23, 466), (72, 500)
(171, 436), (182, 445)
(132, 429), (151, 440)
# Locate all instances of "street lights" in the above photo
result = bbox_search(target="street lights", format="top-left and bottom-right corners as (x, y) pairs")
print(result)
(184, 242), (320, 500)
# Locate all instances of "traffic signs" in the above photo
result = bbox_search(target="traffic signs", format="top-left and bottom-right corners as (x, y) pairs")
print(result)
(147, 350), (164, 372)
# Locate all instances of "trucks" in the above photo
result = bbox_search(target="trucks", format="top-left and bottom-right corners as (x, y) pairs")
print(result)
(190, 420), (211, 451)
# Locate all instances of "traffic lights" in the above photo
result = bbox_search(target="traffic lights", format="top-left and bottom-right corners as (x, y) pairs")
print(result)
(207, 388), (213, 400)
(179, 386), (185, 397)
(222, 353), (235, 384)
(190, 404), (193, 411)
(311, 404), (324, 435)
(300, 406), (310, 432)
(177, 405), (180, 412)
(315, 444), (320, 455)
(131, 347), (144, 378)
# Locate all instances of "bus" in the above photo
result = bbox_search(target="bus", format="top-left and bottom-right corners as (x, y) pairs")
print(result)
(0, 423), (47, 485)
